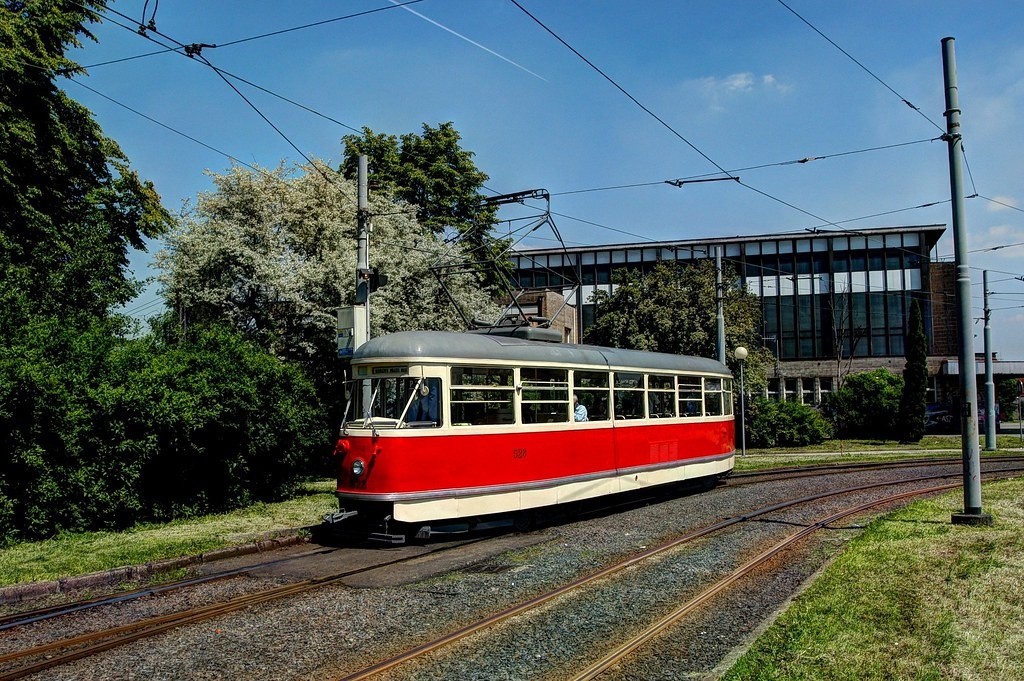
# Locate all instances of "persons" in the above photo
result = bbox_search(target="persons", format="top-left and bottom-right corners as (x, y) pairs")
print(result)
(573, 394), (587, 422)
(632, 382), (660, 414)
(407, 383), (428, 423)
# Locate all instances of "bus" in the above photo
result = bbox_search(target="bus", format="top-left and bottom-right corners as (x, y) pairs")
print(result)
(333, 329), (736, 544)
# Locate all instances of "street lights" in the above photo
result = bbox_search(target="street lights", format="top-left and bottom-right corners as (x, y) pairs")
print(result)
(735, 347), (747, 457)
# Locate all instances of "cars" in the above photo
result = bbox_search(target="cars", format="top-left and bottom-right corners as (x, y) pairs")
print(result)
(923, 400), (1001, 434)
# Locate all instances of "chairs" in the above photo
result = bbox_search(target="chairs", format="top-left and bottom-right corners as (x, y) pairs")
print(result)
(535, 411), (715, 423)
